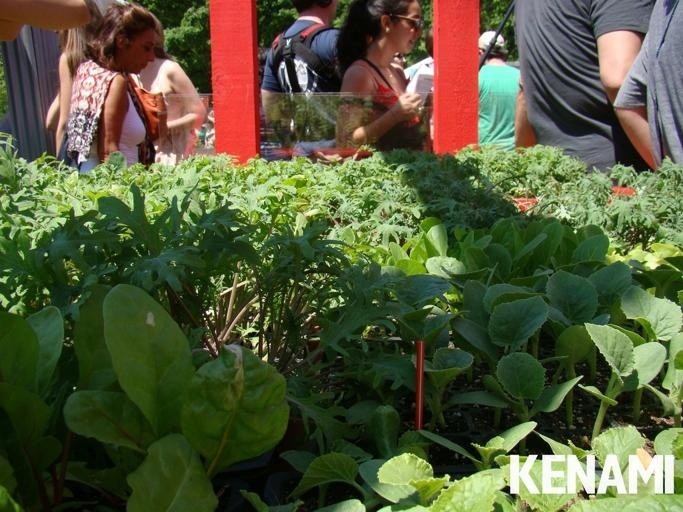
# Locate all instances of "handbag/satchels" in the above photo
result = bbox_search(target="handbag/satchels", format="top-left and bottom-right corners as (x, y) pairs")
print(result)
(129, 74), (168, 141)
(374, 123), (424, 154)
(163, 91), (209, 131)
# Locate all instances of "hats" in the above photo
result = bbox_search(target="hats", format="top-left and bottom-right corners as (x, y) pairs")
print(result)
(478, 31), (504, 50)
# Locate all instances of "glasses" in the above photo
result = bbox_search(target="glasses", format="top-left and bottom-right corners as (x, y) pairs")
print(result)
(391, 13), (425, 30)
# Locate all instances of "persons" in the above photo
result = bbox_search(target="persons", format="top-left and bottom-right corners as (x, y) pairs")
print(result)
(0, 0), (91, 43)
(512, 1), (657, 170)
(45, 1), (103, 163)
(261, 0), (520, 161)
(613, 0), (682, 172)
(129, 19), (205, 166)
(57, 4), (156, 176)
